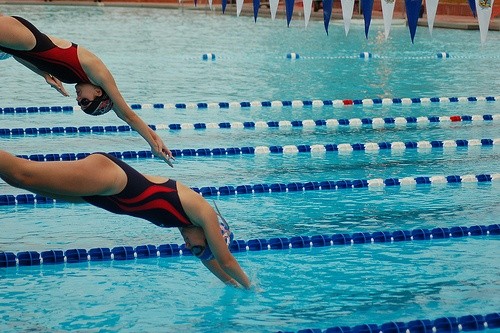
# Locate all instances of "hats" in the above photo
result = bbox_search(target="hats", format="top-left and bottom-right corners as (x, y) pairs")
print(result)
(82, 92), (113, 115)
(194, 222), (233, 260)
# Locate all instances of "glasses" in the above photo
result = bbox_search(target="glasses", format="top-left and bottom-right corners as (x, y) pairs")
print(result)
(77, 97), (109, 106)
(191, 245), (208, 256)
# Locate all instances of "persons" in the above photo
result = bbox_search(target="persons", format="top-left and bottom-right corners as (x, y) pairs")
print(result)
(0, 51), (70, 97)
(0, 14), (176, 168)
(1, 149), (251, 292)
